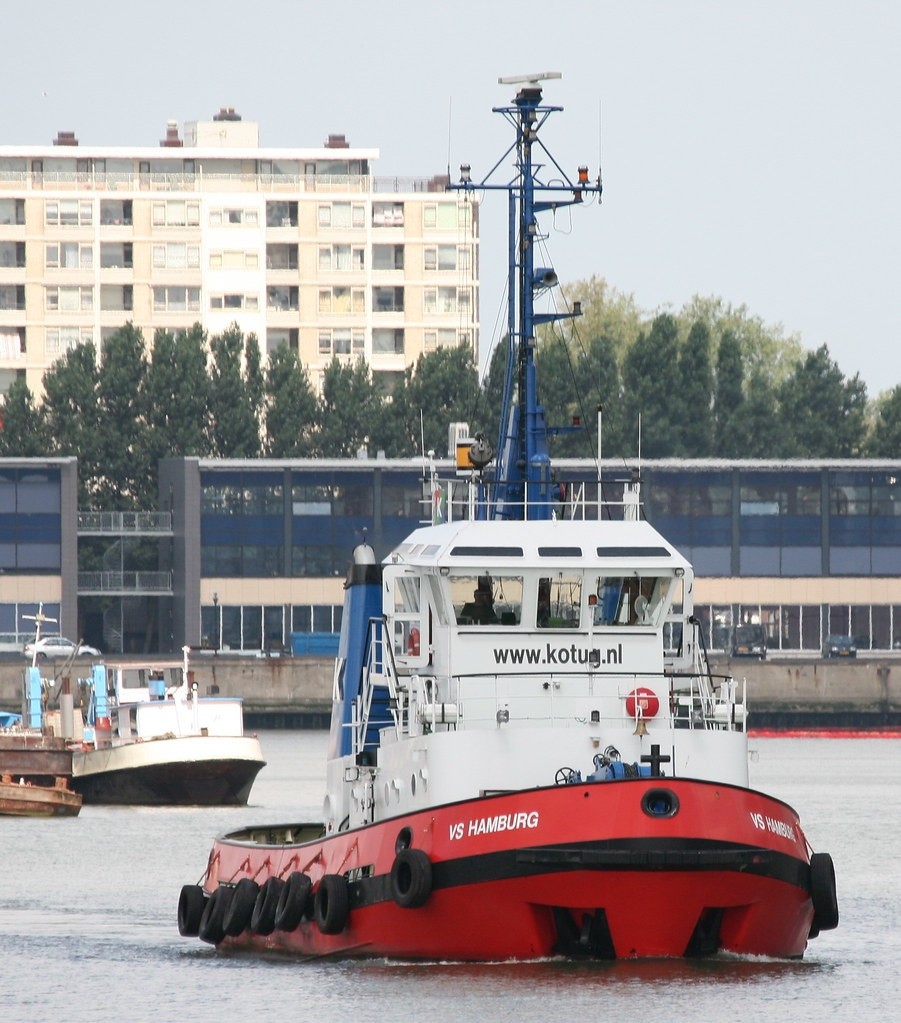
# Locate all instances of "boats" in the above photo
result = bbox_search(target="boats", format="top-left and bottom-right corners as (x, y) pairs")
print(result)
(67, 644), (268, 809)
(176, 70), (841, 984)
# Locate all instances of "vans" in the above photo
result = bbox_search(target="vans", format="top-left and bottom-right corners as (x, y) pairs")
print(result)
(730, 623), (770, 662)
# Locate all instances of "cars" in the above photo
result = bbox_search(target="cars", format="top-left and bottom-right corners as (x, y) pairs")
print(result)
(24, 636), (102, 662)
(822, 633), (858, 660)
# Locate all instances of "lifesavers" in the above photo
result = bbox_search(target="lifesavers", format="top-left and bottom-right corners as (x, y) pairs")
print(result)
(388, 850), (433, 908)
(177, 874), (349, 945)
(407, 628), (421, 658)
(807, 852), (840, 938)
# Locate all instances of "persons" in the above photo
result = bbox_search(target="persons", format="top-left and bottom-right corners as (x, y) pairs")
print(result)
(458, 588), (498, 625)
